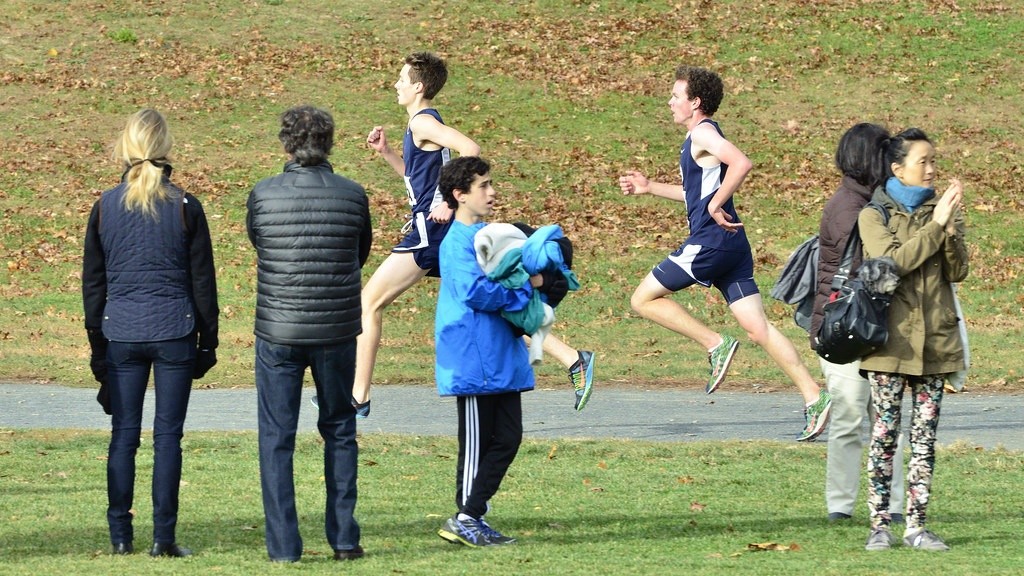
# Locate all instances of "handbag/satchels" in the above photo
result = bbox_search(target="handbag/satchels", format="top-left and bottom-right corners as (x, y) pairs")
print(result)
(814, 200), (892, 366)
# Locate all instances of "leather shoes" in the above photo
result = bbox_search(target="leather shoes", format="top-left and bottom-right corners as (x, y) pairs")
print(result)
(112, 543), (133, 555)
(152, 540), (191, 558)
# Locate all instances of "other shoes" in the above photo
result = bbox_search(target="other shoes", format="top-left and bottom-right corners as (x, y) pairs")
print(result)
(334, 547), (363, 560)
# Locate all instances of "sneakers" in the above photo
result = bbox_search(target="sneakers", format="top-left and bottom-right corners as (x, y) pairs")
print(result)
(437, 509), (517, 548)
(866, 523), (891, 550)
(705, 334), (740, 395)
(794, 387), (832, 443)
(312, 390), (371, 420)
(901, 526), (948, 552)
(568, 348), (595, 410)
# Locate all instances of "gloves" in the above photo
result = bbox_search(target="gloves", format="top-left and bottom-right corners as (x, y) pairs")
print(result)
(91, 355), (107, 386)
(191, 347), (219, 381)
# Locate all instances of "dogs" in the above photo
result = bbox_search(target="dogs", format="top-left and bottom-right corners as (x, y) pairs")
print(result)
(854, 256), (901, 295)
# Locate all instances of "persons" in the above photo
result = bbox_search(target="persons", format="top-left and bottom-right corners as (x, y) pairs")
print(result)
(811, 123), (893, 527)
(858, 126), (969, 553)
(619, 67), (831, 441)
(83, 108), (221, 558)
(245, 105), (372, 560)
(310, 52), (596, 418)
(432, 156), (544, 548)
